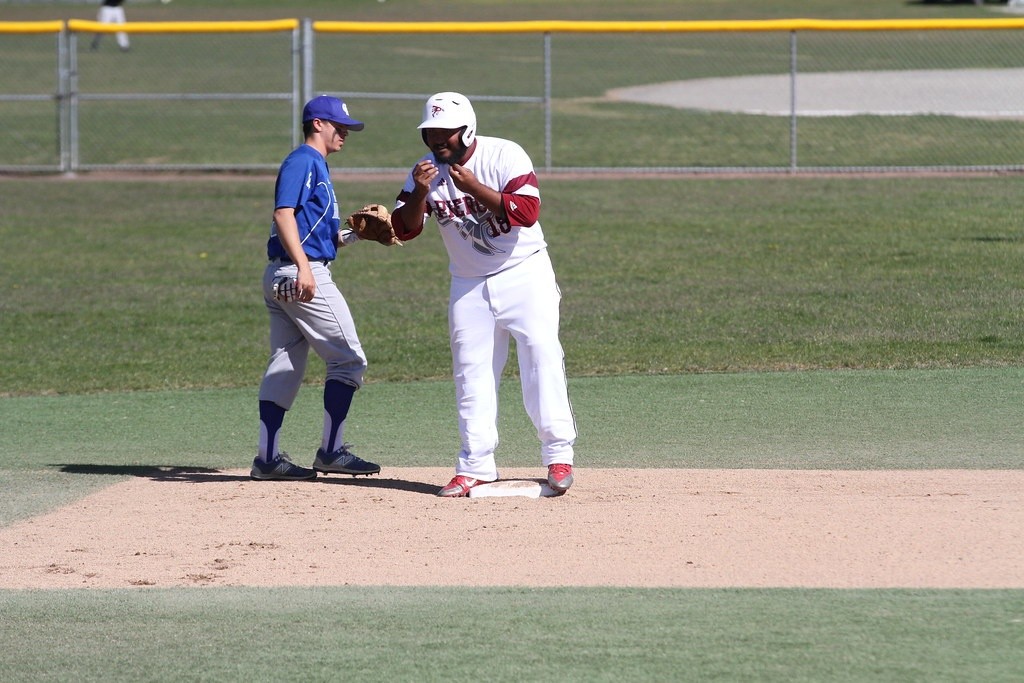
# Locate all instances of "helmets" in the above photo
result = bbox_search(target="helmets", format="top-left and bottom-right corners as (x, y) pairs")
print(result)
(417, 91), (476, 148)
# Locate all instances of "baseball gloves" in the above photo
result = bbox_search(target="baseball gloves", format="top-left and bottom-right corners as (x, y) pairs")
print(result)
(342, 203), (404, 248)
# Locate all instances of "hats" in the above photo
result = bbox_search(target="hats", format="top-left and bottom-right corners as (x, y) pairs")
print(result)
(303, 94), (364, 132)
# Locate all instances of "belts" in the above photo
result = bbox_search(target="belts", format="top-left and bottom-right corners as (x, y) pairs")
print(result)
(272, 257), (323, 263)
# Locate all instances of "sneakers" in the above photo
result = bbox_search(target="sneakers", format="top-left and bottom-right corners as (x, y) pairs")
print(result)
(437, 475), (498, 497)
(250, 453), (317, 481)
(548, 464), (573, 490)
(313, 445), (380, 477)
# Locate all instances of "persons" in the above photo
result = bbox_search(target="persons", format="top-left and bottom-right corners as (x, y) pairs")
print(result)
(249, 95), (402, 480)
(391, 92), (578, 496)
(89, 0), (130, 52)
